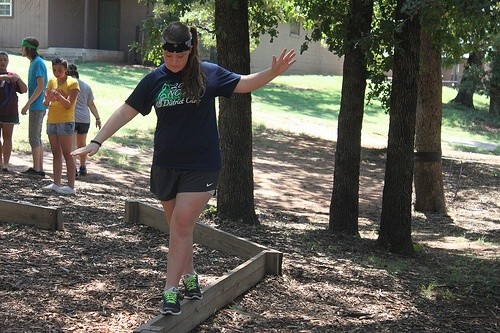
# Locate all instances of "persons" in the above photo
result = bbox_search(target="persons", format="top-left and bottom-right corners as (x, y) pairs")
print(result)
(42, 56), (81, 195)
(0, 51), (27, 172)
(65, 63), (101, 178)
(69, 22), (297, 316)
(18, 37), (47, 178)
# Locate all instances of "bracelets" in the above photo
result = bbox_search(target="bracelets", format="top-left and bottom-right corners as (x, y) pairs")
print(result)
(91, 140), (101, 147)
(96, 118), (100, 120)
(30, 96), (36, 101)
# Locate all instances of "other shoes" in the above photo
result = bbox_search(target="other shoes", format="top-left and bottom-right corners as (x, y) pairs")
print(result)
(79, 166), (87, 176)
(17, 168), (45, 177)
(1, 167), (10, 173)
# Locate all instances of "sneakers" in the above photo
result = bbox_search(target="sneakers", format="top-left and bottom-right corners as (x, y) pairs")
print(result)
(43, 184), (60, 191)
(162, 289), (182, 315)
(181, 272), (203, 299)
(55, 186), (75, 195)
